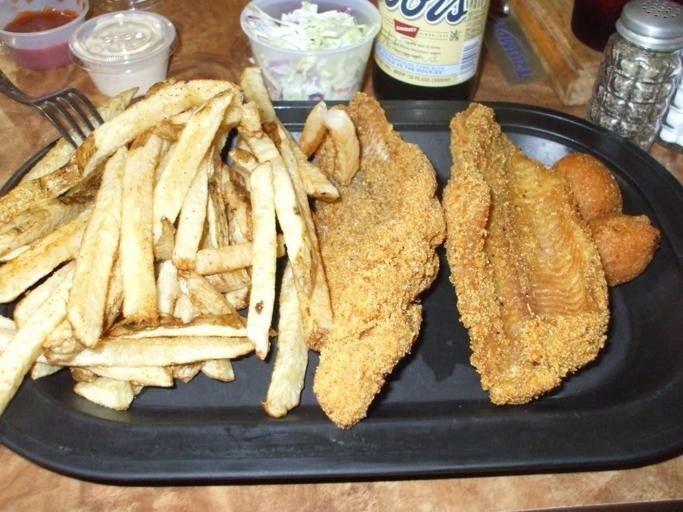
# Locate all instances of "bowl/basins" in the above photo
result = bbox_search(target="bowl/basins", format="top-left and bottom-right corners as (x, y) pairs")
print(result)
(0, 0), (91, 71)
(68, 9), (179, 99)
(239, 0), (383, 101)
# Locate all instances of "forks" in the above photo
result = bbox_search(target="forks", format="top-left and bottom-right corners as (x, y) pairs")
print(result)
(0, 67), (106, 151)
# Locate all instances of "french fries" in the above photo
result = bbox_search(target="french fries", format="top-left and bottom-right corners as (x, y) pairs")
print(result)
(0, 66), (361, 419)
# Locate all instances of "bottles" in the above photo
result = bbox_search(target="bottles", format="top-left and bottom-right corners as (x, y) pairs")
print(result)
(584, 0), (683, 153)
(372, 0), (491, 103)
